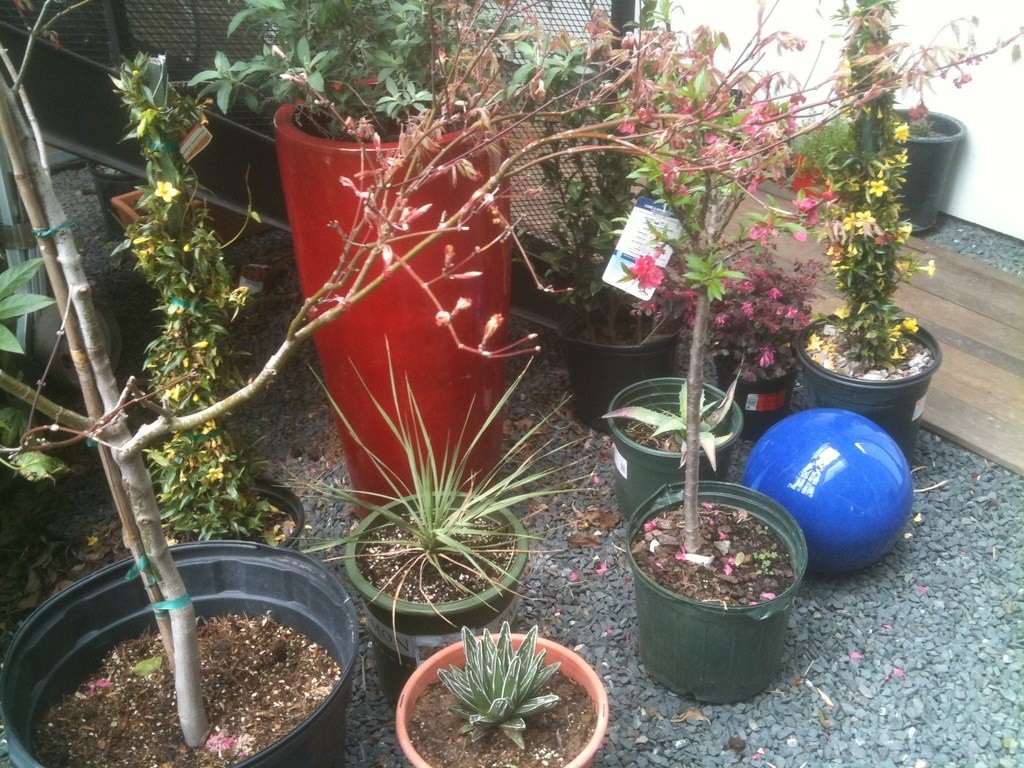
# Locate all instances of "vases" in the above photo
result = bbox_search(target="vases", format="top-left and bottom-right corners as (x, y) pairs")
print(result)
(713, 351), (800, 441)
(623, 480), (808, 705)
(0, 541), (362, 767)
(794, 309), (943, 473)
(167, 474), (307, 549)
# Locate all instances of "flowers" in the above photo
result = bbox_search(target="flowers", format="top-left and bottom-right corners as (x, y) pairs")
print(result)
(814, 0), (936, 363)
(108, 50), (276, 542)
(634, 258), (819, 368)
(614, 28), (840, 309)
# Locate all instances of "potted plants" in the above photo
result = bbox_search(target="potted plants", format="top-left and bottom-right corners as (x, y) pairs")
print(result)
(292, 336), (599, 713)
(395, 621), (608, 767)
(888, 101), (968, 234)
(184, 0), (591, 532)
(599, 364), (744, 523)
